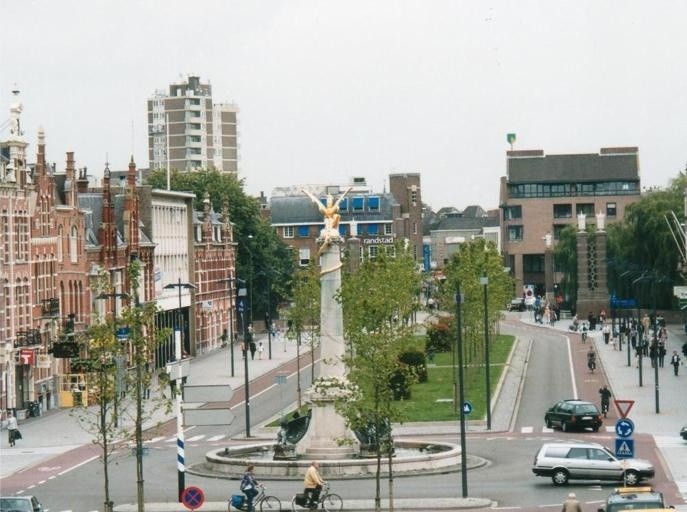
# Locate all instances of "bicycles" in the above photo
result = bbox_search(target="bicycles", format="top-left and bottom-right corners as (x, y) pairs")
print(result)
(291, 480), (343, 512)
(567, 321), (586, 335)
(589, 358), (596, 373)
(226, 483), (281, 511)
(583, 332), (587, 343)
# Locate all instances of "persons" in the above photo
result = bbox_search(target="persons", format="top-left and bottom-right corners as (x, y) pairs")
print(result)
(597, 384), (611, 413)
(300, 187), (353, 237)
(532, 295), (683, 377)
(239, 465), (266, 511)
(240, 322), (297, 361)
(3, 410), (18, 448)
(303, 461), (327, 510)
(141, 369), (152, 399)
(157, 367), (169, 399)
(561, 491), (582, 512)
(168, 380), (177, 399)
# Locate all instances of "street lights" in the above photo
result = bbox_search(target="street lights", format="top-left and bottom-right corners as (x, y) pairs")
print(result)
(236, 281), (252, 438)
(161, 276), (200, 358)
(213, 276), (242, 377)
(95, 286), (136, 429)
(600, 256), (660, 413)
(479, 273), (491, 429)
(452, 292), (469, 497)
(250, 271), (267, 327)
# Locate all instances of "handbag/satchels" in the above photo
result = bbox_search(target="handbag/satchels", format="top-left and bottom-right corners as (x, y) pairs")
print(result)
(231, 495), (245, 508)
(295, 493), (306, 505)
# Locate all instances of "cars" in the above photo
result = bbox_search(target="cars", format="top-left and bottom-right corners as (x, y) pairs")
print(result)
(678, 424), (686, 439)
(596, 485), (678, 511)
(614, 506), (680, 512)
(507, 297), (525, 313)
(0, 495), (44, 512)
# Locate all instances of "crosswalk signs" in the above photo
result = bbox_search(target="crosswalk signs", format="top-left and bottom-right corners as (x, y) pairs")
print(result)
(613, 438), (636, 458)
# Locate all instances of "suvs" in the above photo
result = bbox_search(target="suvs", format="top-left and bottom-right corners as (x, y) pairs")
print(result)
(532, 442), (657, 487)
(542, 398), (603, 433)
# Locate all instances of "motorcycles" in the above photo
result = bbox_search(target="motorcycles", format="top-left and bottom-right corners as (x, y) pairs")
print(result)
(23, 401), (40, 418)
